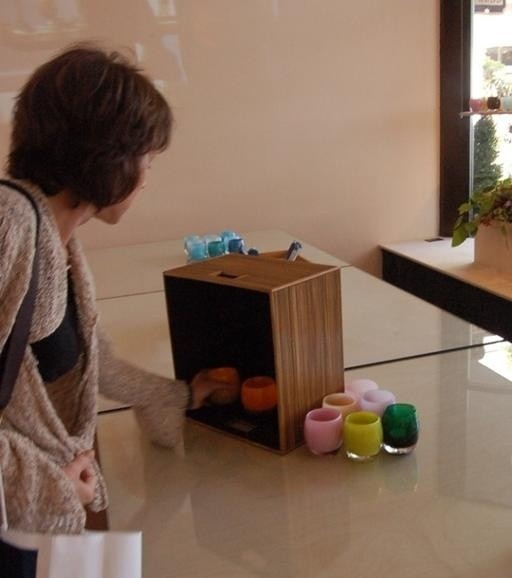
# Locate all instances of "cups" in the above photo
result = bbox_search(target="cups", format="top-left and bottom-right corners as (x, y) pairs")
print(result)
(303, 407), (344, 456)
(321, 378), (396, 413)
(241, 375), (278, 415)
(210, 365), (239, 407)
(382, 402), (419, 455)
(343, 411), (383, 462)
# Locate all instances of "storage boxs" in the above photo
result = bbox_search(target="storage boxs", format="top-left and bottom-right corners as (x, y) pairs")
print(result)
(164, 252), (346, 455)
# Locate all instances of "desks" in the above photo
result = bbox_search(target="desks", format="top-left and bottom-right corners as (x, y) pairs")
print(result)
(95, 264), (504, 413)
(382, 237), (512, 339)
(93, 340), (511, 576)
(81, 229), (350, 299)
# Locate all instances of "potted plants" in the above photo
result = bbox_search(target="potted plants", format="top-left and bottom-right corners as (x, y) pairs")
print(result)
(452, 174), (512, 270)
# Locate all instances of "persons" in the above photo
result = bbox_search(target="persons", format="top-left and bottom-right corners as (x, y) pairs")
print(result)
(0, 43), (233, 578)
